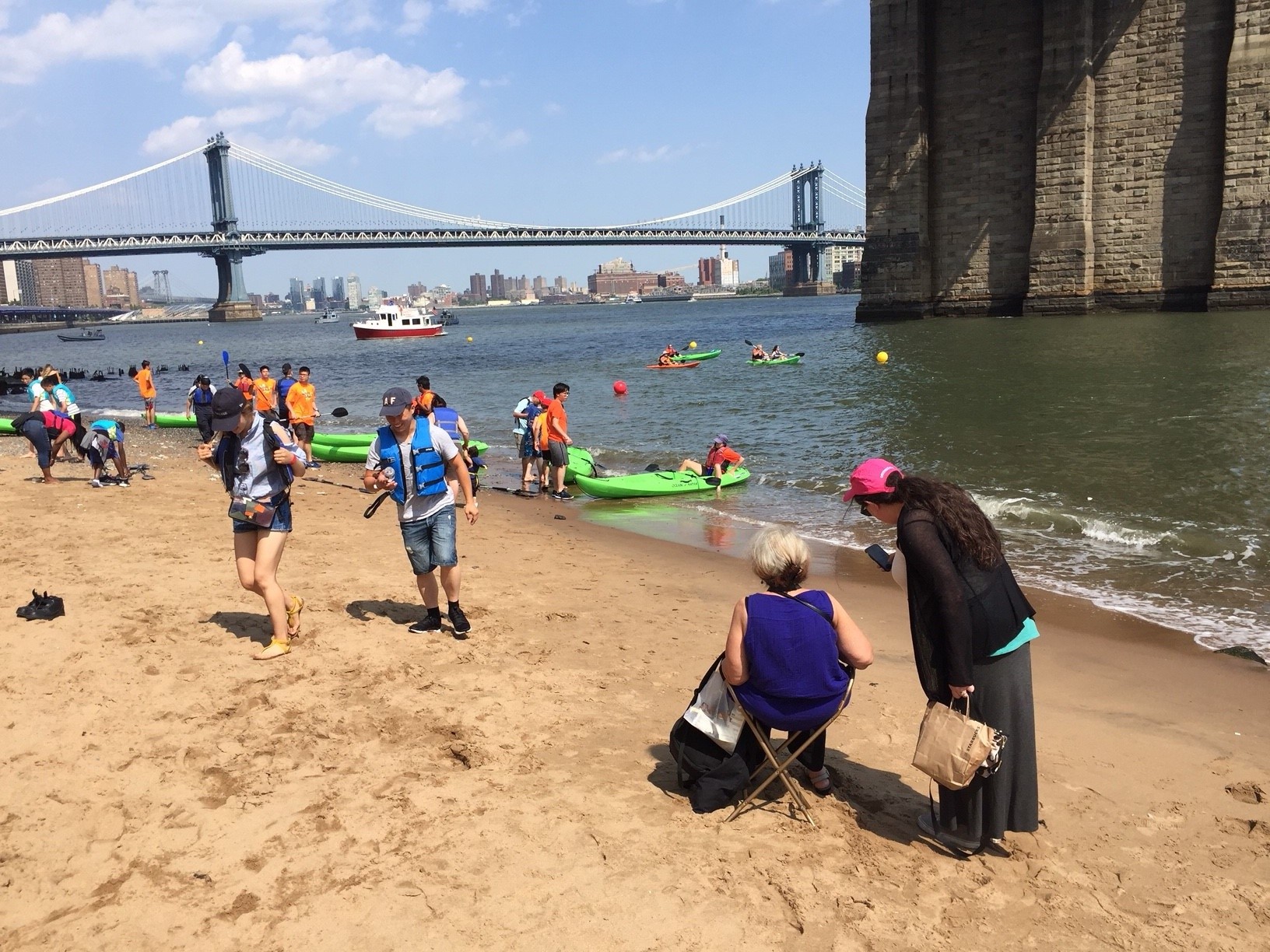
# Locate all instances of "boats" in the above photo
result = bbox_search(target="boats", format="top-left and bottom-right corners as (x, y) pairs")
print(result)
(57, 327), (106, 342)
(349, 292), (460, 341)
(745, 355), (801, 365)
(646, 360), (701, 369)
(141, 412), (198, 428)
(547, 444), (596, 485)
(314, 306), (340, 324)
(573, 463), (752, 500)
(311, 433), (489, 455)
(670, 348), (722, 361)
(310, 442), (370, 463)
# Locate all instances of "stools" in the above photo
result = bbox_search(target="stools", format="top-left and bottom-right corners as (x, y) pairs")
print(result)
(723, 665), (857, 831)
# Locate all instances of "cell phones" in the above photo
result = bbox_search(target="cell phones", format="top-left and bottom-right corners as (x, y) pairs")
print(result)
(864, 544), (893, 572)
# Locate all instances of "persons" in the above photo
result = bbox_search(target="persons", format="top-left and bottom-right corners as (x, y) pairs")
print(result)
(197, 386), (307, 661)
(363, 387), (478, 635)
(721, 528), (874, 794)
(10, 344), (788, 501)
(843, 458), (1042, 850)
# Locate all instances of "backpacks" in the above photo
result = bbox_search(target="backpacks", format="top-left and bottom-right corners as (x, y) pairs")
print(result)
(670, 651), (751, 810)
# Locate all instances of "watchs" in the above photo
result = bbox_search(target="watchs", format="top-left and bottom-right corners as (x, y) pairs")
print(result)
(315, 409), (319, 412)
(373, 480), (380, 491)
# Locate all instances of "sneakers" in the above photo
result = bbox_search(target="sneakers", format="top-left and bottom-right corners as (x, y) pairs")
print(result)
(551, 490), (575, 499)
(448, 605), (472, 634)
(92, 474), (130, 487)
(16, 588), (44, 617)
(408, 616), (442, 634)
(26, 591), (65, 620)
(305, 460), (321, 470)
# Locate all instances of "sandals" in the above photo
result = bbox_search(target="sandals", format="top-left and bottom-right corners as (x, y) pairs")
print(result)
(802, 765), (832, 794)
(286, 595), (304, 639)
(253, 635), (292, 660)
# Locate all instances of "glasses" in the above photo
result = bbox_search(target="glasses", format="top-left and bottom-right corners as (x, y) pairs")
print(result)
(238, 448), (249, 472)
(299, 373), (309, 377)
(562, 392), (570, 395)
(860, 499), (874, 517)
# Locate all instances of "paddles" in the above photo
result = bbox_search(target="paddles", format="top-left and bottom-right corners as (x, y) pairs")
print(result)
(222, 350), (229, 388)
(568, 453), (608, 471)
(775, 353), (805, 357)
(238, 363), (281, 417)
(658, 359), (686, 366)
(645, 464), (721, 486)
(479, 485), (540, 495)
(745, 339), (767, 355)
(302, 476), (377, 495)
(670, 345), (689, 356)
(128, 464), (155, 480)
(264, 407), (348, 423)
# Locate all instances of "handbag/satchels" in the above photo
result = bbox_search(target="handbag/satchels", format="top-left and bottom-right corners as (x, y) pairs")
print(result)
(683, 669), (745, 757)
(228, 496), (277, 529)
(911, 694), (994, 791)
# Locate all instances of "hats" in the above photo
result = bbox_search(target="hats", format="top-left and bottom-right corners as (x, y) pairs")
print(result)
(843, 459), (904, 501)
(238, 370), (244, 374)
(212, 387), (246, 431)
(282, 363), (291, 372)
(379, 388), (413, 416)
(533, 390), (552, 406)
(713, 435), (728, 444)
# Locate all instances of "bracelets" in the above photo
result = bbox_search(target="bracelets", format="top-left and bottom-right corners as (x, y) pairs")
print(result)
(289, 454), (296, 465)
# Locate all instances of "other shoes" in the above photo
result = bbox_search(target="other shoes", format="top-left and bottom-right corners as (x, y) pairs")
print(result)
(982, 827), (1004, 844)
(147, 423), (159, 430)
(917, 814), (981, 849)
